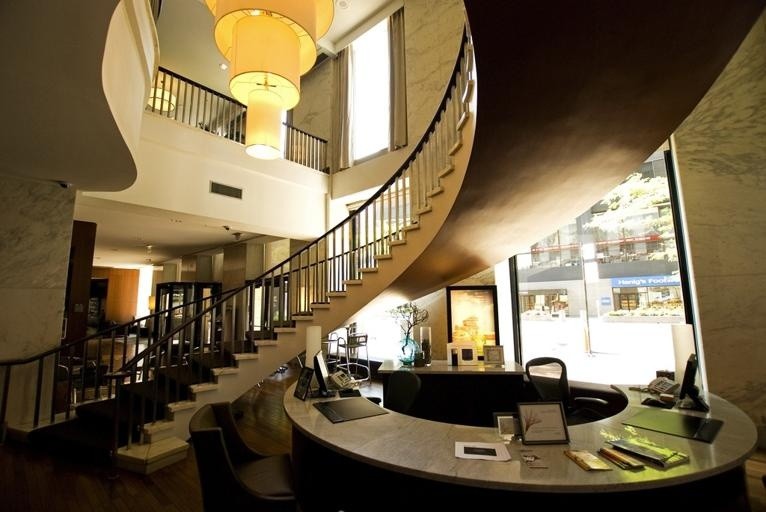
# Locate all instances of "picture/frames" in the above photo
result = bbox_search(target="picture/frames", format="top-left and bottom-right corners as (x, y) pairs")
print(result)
(516, 401), (571, 445)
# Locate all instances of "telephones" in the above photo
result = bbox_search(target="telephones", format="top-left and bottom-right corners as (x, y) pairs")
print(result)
(647, 376), (681, 396)
(330, 369), (351, 388)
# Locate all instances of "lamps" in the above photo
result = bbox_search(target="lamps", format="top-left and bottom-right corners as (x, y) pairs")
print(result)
(205, 0), (335, 161)
(147, 88), (177, 113)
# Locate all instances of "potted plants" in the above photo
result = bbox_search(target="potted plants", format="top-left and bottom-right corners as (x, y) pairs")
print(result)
(387, 302), (429, 366)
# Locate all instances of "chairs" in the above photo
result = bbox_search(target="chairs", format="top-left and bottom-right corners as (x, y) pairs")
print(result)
(385, 371), (422, 413)
(70, 364), (108, 404)
(188, 401), (298, 511)
(525, 357), (609, 425)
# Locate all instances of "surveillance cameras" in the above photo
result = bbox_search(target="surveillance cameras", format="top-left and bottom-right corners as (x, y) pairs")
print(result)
(60, 183), (71, 188)
(224, 225), (231, 230)
(235, 233), (242, 240)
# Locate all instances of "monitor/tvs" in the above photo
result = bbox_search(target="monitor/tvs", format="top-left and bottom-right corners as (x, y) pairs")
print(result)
(677, 353), (709, 412)
(308, 350), (336, 398)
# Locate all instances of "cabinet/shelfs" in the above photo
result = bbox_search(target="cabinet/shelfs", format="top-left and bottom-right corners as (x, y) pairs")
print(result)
(319, 331), (372, 387)
(151, 281), (222, 366)
